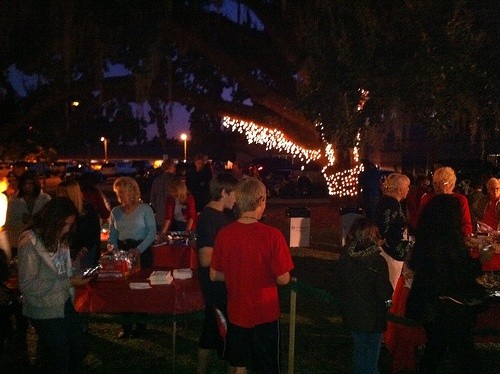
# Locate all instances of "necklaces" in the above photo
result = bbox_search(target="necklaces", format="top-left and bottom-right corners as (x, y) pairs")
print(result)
(240, 217), (258, 222)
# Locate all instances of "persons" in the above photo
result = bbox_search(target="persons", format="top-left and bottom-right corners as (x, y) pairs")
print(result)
(415, 167), (472, 237)
(333, 217), (394, 374)
(405, 194), (487, 374)
(161, 181), (197, 231)
(197, 173), (239, 360)
(359, 160), (379, 219)
(210, 177), (295, 374)
(188, 155), (212, 210)
(109, 178), (156, 336)
(19, 197), (82, 374)
(3, 162), (28, 195)
(373, 173), (410, 261)
(471, 178), (500, 232)
(57, 181), (101, 267)
(0, 170), (50, 261)
(231, 161), (249, 180)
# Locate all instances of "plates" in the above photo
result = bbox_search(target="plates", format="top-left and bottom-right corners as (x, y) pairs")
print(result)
(148, 271), (174, 285)
(173, 268), (193, 279)
(130, 282), (151, 289)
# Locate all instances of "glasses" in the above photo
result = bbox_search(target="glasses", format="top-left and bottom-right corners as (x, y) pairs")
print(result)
(21, 181), (34, 187)
(487, 187), (499, 192)
(9, 175), (16, 178)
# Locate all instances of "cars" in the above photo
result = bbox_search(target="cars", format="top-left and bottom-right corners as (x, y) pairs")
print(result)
(0, 159), (152, 184)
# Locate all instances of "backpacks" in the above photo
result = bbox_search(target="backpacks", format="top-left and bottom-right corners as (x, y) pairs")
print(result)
(358, 270), (394, 315)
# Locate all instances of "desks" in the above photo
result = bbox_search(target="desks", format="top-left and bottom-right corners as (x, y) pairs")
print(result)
(10, 263), (206, 374)
(151, 233), (198, 271)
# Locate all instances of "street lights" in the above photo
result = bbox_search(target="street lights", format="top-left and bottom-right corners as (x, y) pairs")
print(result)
(100, 137), (107, 159)
(180, 132), (189, 160)
